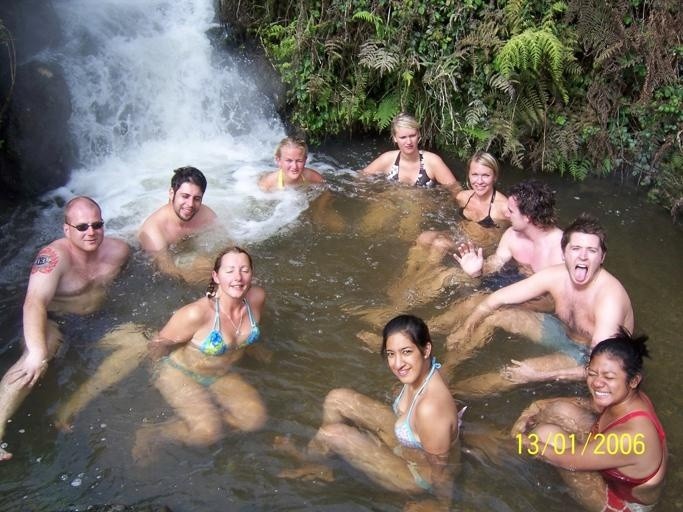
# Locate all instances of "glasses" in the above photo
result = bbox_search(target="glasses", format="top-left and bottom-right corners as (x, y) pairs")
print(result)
(65, 219), (104, 232)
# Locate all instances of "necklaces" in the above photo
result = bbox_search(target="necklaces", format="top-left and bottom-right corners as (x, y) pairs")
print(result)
(218, 302), (243, 337)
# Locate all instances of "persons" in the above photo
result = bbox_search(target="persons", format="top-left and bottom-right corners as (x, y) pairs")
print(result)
(386, 152), (509, 305)
(437, 218), (636, 402)
(270, 312), (464, 511)
(355, 112), (456, 240)
(133, 247), (267, 465)
(340, 179), (565, 355)
(0, 194), (149, 463)
(138, 167), (237, 285)
(257, 134), (344, 234)
(460, 325), (667, 512)
